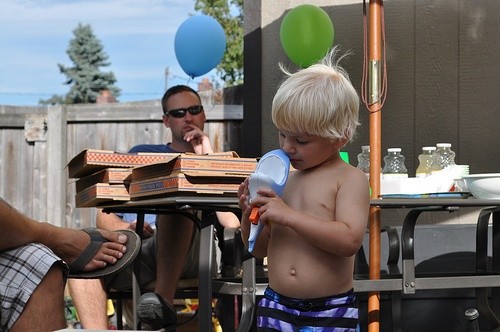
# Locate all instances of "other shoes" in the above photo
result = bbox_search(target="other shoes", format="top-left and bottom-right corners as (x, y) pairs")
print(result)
(137, 292), (181, 332)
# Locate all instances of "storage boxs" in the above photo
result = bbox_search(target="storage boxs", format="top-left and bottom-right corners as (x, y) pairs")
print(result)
(75, 167), (133, 193)
(68, 148), (240, 178)
(130, 173), (242, 197)
(133, 154), (257, 177)
(76, 183), (130, 208)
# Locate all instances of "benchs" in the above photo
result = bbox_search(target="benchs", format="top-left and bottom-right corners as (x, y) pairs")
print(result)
(108, 224), (500, 332)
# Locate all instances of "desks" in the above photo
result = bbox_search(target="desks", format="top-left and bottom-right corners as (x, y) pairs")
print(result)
(102, 196), (500, 332)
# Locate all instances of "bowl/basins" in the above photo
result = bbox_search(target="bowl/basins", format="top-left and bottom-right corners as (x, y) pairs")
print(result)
(460, 174), (500, 200)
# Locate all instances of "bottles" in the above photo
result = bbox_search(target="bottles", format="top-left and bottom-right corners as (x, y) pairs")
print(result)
(415, 147), (441, 177)
(383, 148), (408, 174)
(436, 143), (456, 170)
(356, 146), (371, 172)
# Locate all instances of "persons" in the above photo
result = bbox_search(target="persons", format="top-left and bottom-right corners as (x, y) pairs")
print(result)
(67, 85), (240, 331)
(0, 197), (141, 332)
(239, 44), (370, 331)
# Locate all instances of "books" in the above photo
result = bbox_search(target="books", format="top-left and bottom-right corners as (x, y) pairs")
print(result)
(66, 144), (259, 208)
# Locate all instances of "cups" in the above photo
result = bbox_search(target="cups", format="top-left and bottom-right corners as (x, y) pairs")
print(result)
(432, 165), (470, 192)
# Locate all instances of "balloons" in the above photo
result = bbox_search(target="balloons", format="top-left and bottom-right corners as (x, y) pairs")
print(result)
(280, 5), (334, 68)
(174, 14), (227, 79)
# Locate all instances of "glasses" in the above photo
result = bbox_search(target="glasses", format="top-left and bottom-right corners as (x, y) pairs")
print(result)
(165, 104), (205, 117)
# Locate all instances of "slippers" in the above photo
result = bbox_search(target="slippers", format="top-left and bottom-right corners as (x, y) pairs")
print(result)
(63, 227), (140, 278)
